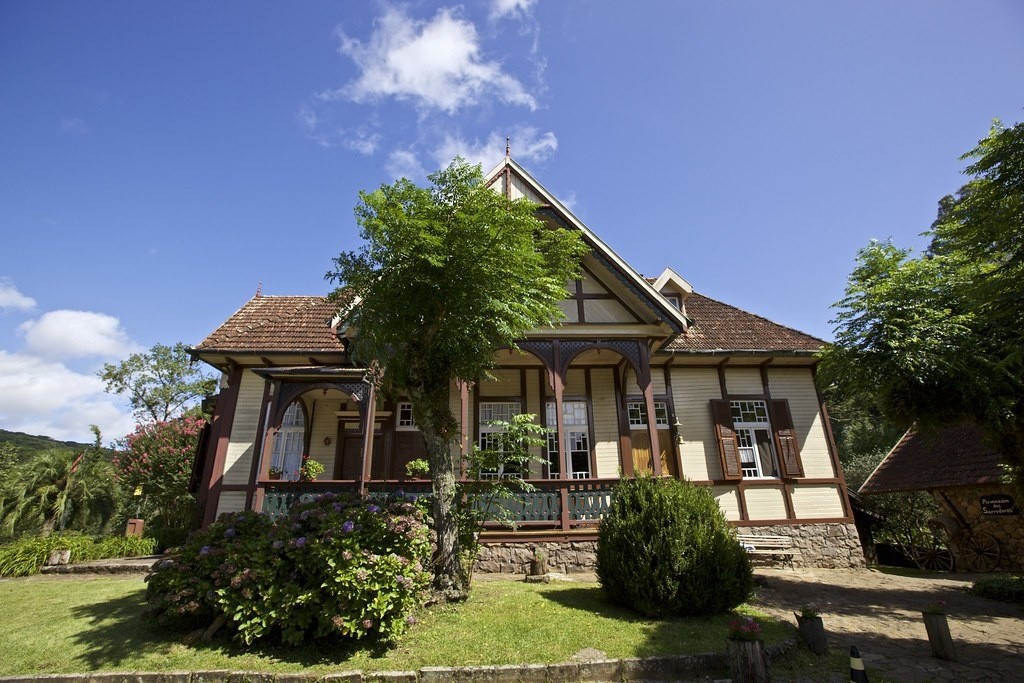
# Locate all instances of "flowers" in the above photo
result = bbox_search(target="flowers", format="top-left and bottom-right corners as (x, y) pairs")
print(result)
(293, 456), (325, 480)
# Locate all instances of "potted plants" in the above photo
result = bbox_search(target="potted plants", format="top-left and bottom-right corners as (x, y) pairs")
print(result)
(269, 466), (287, 480)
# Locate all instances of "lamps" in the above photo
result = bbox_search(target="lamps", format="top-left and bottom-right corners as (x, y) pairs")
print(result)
(670, 414), (682, 426)
